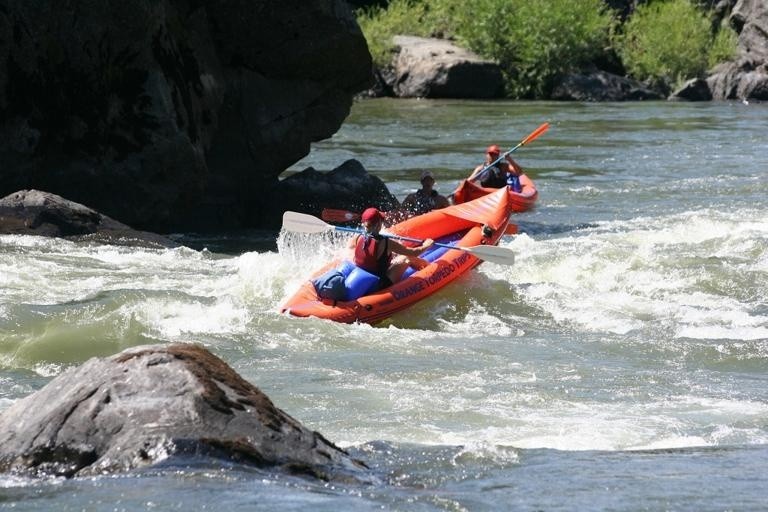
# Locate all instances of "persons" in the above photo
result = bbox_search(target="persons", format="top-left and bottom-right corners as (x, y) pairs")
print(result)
(348, 207), (435, 289)
(400, 168), (449, 218)
(455, 145), (524, 195)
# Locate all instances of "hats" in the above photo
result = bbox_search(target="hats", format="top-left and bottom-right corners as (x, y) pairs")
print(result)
(361, 208), (386, 226)
(420, 170), (435, 180)
(486, 144), (501, 155)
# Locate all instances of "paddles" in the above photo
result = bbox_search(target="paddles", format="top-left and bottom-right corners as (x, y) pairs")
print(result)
(322, 208), (419, 222)
(283, 211), (514, 265)
(445, 123), (551, 199)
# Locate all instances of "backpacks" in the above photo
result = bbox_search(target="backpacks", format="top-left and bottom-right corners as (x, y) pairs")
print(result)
(310, 270), (346, 303)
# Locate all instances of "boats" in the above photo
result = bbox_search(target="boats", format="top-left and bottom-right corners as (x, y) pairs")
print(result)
(449, 163), (540, 212)
(281, 182), (518, 321)
